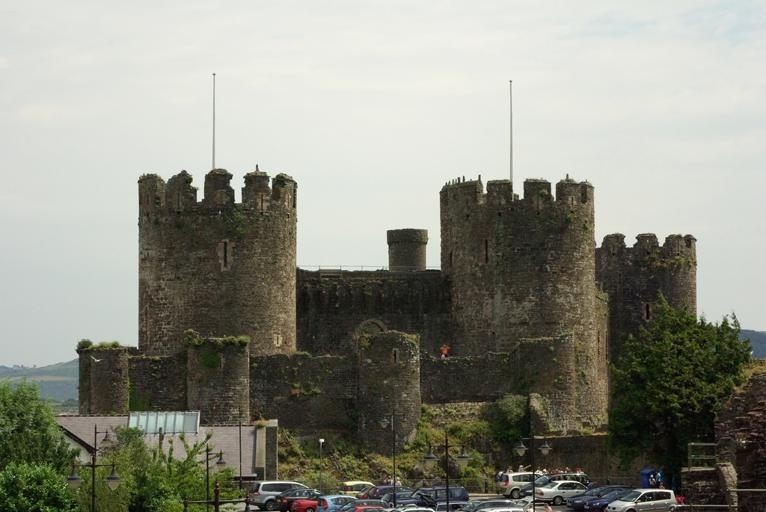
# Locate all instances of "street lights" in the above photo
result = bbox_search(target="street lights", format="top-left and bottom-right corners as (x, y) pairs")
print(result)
(67, 456), (123, 511)
(93, 422), (114, 458)
(518, 431), (553, 511)
(423, 428), (469, 512)
(204, 443), (226, 510)
(319, 438), (324, 490)
(379, 409), (407, 507)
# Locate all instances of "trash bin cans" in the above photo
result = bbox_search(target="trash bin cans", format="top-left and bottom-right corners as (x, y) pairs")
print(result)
(640, 468), (657, 488)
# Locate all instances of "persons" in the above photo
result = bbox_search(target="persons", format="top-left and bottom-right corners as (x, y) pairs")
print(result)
(649, 475), (655, 488)
(495, 464), (583, 482)
(656, 471), (661, 488)
(440, 342), (449, 360)
